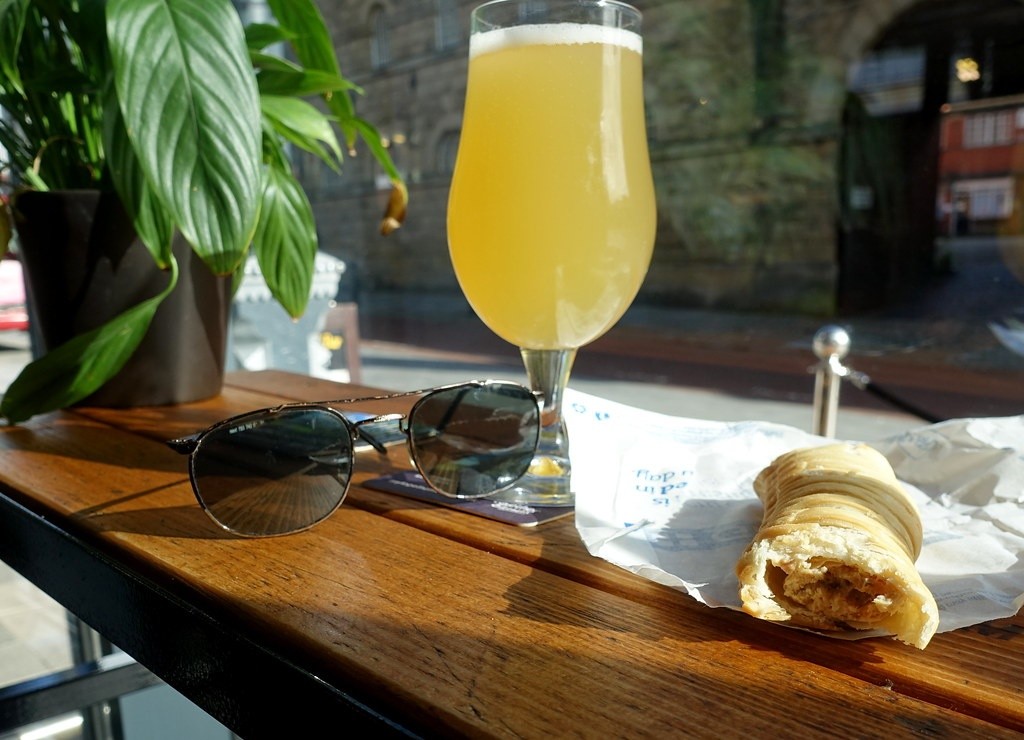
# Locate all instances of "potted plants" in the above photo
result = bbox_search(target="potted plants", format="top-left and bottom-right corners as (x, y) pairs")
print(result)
(0, 0), (408, 433)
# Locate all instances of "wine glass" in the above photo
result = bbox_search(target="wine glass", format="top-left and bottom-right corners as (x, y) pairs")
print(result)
(447, 0), (656, 507)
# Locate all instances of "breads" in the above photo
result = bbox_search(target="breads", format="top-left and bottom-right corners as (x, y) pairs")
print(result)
(736, 442), (940, 651)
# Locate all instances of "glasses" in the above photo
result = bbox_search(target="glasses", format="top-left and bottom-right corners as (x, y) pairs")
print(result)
(166, 380), (546, 539)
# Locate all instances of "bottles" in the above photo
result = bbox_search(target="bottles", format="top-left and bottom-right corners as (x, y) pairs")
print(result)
(831, 92), (901, 315)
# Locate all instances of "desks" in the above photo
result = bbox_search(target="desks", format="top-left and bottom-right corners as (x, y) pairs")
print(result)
(0, 368), (1024, 740)
